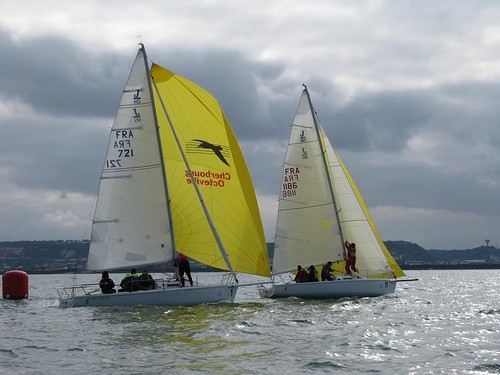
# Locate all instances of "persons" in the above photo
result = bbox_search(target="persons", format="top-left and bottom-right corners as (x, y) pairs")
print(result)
(307, 265), (318, 278)
(174, 253), (193, 287)
(294, 265), (309, 283)
(118, 269), (140, 292)
(139, 270), (155, 289)
(321, 262), (334, 281)
(345, 241), (359, 275)
(99, 271), (116, 294)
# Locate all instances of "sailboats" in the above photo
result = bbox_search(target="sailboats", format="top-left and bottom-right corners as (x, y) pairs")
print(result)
(56, 33), (274, 310)
(257, 82), (421, 300)
